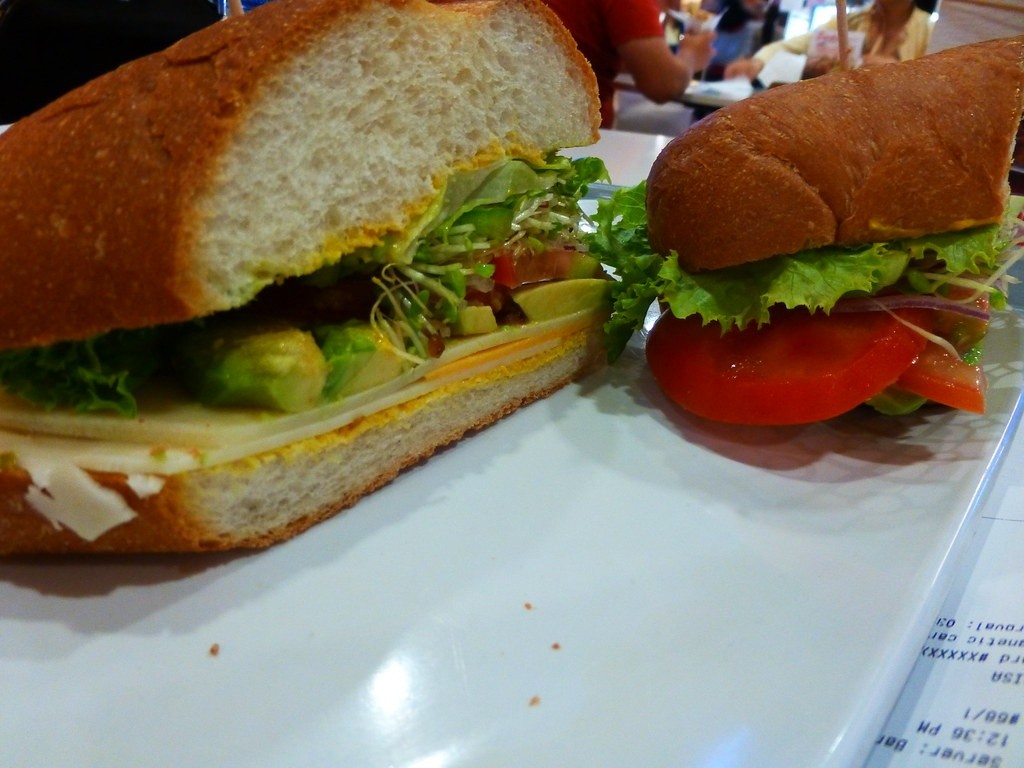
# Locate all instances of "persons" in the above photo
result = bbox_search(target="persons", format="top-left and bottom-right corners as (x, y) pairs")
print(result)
(544, 0), (932, 133)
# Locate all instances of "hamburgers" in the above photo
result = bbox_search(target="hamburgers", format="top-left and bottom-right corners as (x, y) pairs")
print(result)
(587, 31), (1024, 427)
(0, 0), (654, 555)
